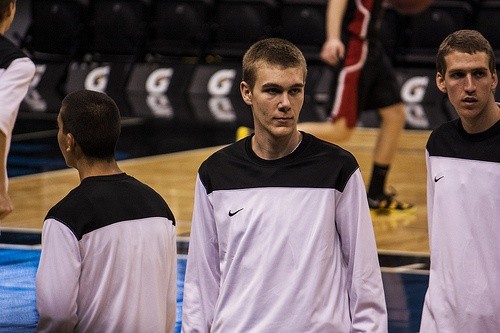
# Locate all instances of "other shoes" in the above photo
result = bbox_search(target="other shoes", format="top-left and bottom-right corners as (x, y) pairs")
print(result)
(363, 188), (417, 212)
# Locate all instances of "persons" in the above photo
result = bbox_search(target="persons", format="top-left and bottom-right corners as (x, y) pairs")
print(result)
(418, 30), (500, 333)
(0, 0), (37, 218)
(34, 90), (177, 333)
(320, 0), (414, 210)
(180, 38), (387, 333)
